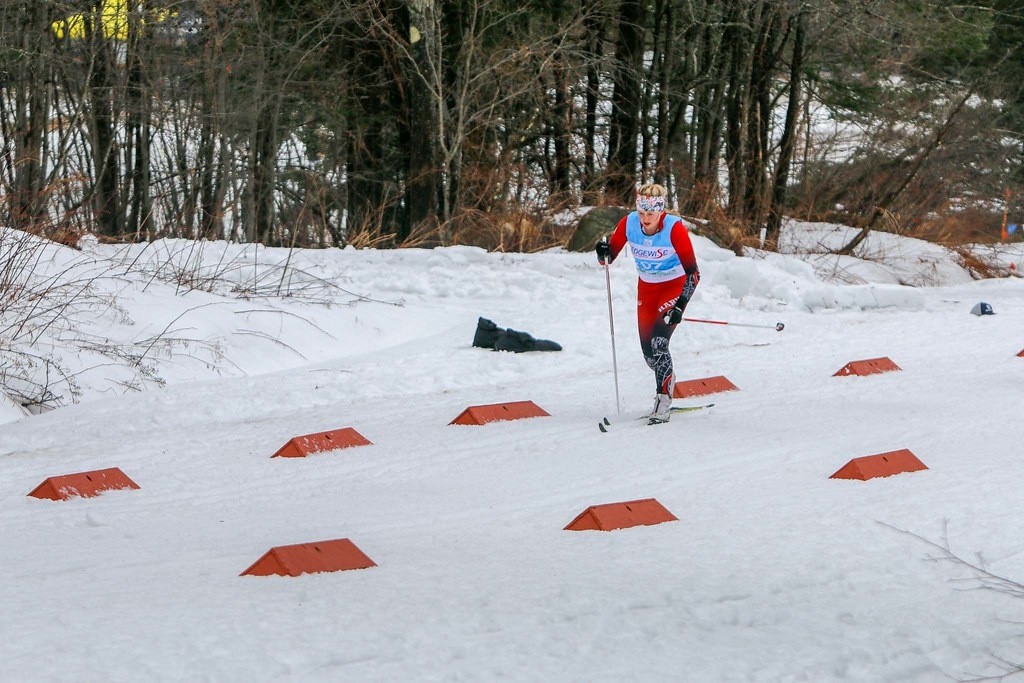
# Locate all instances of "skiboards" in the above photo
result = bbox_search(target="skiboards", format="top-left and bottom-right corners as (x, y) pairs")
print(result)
(599, 404), (716, 433)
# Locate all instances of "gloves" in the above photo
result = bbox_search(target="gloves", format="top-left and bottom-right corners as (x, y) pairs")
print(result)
(670, 295), (688, 323)
(596, 237), (610, 257)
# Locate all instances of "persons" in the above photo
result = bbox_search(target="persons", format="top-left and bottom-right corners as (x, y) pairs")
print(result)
(596, 184), (700, 423)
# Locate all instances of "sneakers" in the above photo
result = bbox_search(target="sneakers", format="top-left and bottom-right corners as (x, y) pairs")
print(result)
(649, 393), (672, 422)
